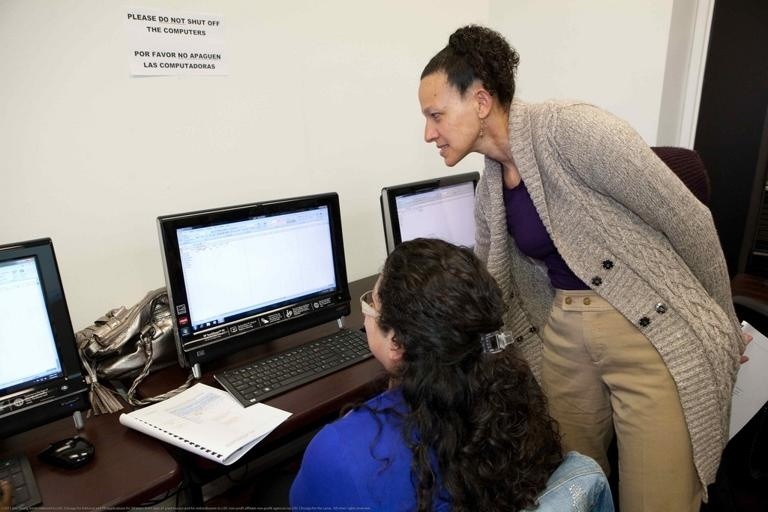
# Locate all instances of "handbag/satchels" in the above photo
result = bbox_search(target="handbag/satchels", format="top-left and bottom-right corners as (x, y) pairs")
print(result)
(76, 287), (181, 384)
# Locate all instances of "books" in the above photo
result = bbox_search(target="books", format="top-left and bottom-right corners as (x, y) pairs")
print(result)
(119, 381), (294, 466)
(728, 320), (768, 442)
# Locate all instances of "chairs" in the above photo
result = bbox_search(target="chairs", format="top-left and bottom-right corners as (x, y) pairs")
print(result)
(519, 452), (616, 512)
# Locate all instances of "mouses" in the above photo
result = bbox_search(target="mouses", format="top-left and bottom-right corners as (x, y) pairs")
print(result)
(36, 435), (96, 469)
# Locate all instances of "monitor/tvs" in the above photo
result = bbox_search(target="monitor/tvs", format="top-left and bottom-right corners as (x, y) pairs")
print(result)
(0, 239), (94, 437)
(379, 169), (486, 262)
(155, 191), (352, 380)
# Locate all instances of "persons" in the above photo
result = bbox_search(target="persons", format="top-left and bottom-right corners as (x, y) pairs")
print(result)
(419, 23), (752, 512)
(289, 239), (566, 512)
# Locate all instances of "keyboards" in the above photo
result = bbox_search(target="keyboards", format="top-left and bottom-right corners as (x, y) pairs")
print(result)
(212, 327), (374, 407)
(0, 451), (43, 511)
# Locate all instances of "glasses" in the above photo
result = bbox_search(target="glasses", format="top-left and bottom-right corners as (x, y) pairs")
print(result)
(359, 291), (385, 316)
